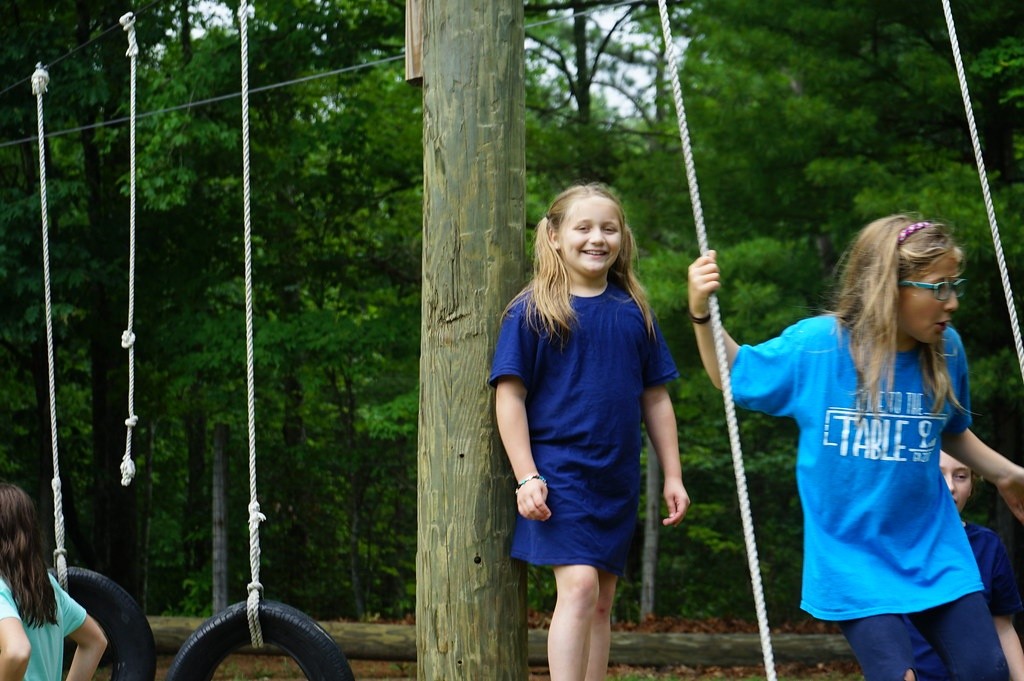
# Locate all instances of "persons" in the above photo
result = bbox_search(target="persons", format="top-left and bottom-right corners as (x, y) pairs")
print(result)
(686, 214), (1024, 681)
(0, 483), (107, 681)
(486, 183), (692, 681)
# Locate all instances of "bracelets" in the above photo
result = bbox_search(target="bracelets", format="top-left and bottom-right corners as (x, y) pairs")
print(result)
(688, 312), (711, 324)
(515, 473), (548, 495)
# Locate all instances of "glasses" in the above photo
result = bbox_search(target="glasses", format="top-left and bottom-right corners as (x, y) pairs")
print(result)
(899, 279), (969, 302)
